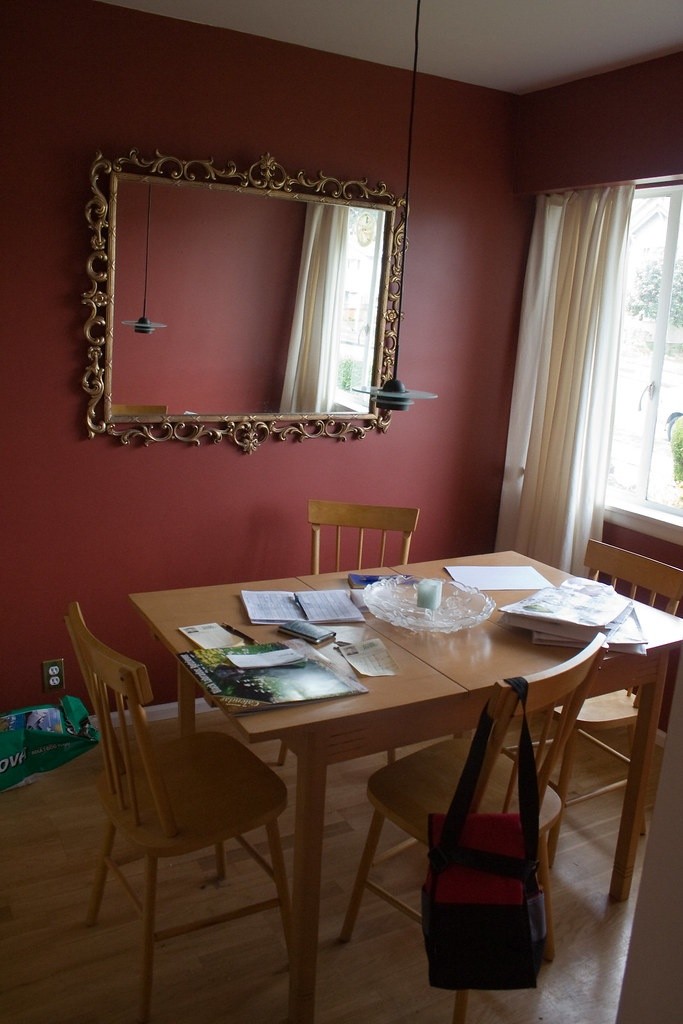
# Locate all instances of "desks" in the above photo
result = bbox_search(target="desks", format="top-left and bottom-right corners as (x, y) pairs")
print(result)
(129, 548), (683, 1023)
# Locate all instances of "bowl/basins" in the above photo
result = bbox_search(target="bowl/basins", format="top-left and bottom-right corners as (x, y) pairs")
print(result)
(360, 575), (497, 633)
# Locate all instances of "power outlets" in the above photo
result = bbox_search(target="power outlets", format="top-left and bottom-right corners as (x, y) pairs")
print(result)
(43, 658), (65, 692)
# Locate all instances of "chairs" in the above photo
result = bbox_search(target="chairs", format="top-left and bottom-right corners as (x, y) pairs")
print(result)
(63, 599), (290, 1024)
(495, 536), (683, 833)
(278, 501), (420, 771)
(336, 631), (617, 1021)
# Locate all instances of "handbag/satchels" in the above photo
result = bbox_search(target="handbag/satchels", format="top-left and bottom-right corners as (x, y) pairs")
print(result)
(422, 677), (546, 990)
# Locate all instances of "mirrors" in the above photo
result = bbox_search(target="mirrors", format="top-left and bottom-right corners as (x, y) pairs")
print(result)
(79, 149), (409, 453)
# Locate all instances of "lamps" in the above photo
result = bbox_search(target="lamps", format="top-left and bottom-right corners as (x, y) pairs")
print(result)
(121, 184), (168, 335)
(355, 1), (438, 411)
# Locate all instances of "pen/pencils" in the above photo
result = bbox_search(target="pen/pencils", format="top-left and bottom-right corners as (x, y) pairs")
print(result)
(294, 592), (310, 620)
(222, 622), (260, 645)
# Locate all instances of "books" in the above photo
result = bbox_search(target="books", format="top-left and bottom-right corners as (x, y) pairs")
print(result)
(177, 639), (369, 716)
(241, 589), (366, 625)
(278, 620), (336, 644)
(348, 573), (418, 588)
(499, 584), (648, 655)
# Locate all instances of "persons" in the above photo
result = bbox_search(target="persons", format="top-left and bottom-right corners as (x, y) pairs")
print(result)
(347, 647), (358, 655)
(188, 628), (198, 634)
